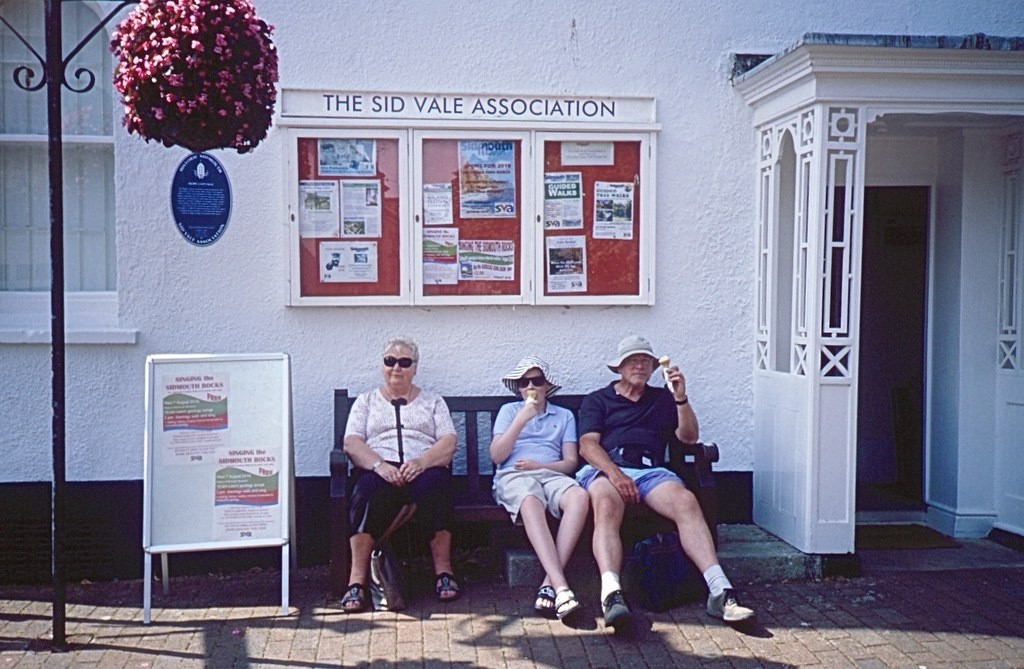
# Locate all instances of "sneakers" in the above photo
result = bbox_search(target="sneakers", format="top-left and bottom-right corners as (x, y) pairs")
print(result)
(603, 589), (637, 637)
(704, 590), (756, 626)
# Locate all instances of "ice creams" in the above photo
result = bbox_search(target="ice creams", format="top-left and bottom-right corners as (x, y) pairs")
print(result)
(659, 355), (671, 370)
(527, 390), (539, 399)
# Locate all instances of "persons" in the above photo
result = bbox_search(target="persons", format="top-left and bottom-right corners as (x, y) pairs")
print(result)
(340, 335), (463, 612)
(573, 335), (757, 626)
(490, 355), (591, 622)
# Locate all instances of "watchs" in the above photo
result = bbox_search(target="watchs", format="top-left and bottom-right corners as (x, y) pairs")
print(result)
(370, 460), (386, 472)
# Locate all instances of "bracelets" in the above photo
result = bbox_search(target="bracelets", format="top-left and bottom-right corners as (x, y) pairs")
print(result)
(676, 395), (690, 405)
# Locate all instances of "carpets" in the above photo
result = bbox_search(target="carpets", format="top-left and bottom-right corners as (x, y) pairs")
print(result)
(854, 524), (964, 551)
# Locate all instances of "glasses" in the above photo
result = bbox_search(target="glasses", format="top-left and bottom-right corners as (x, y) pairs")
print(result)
(516, 376), (545, 388)
(382, 356), (417, 368)
(626, 356), (652, 367)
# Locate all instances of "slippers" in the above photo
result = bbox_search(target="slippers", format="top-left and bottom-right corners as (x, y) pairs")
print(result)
(555, 589), (581, 619)
(340, 582), (371, 611)
(433, 572), (460, 602)
(533, 585), (555, 613)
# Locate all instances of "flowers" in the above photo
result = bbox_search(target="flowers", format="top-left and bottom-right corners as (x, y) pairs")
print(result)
(108, 0), (279, 155)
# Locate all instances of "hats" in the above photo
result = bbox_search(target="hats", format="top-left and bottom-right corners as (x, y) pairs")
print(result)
(605, 335), (660, 372)
(502, 355), (562, 397)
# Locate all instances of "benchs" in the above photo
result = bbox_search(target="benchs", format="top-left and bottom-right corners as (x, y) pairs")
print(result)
(330, 389), (721, 611)
